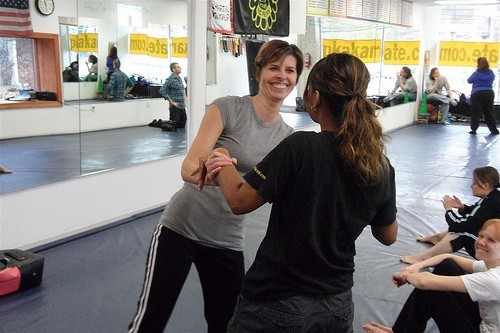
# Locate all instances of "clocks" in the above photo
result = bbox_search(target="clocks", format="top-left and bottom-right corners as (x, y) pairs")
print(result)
(35, 0), (55, 16)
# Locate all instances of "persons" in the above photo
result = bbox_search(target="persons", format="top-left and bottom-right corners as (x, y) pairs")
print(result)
(466, 57), (500, 135)
(425, 68), (452, 125)
(400, 166), (500, 265)
(192, 55), (397, 333)
(63, 62), (80, 82)
(364, 219), (500, 333)
(383, 67), (417, 107)
(126, 41), (301, 333)
(149, 62), (186, 131)
(104, 45), (120, 84)
(106, 60), (127, 101)
(84, 55), (97, 82)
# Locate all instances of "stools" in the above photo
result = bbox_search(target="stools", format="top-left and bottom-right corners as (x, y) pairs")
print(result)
(427, 99), (442, 124)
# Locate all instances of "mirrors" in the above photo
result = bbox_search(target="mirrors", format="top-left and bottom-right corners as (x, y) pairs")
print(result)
(0, 0), (192, 195)
(320, 18), (418, 111)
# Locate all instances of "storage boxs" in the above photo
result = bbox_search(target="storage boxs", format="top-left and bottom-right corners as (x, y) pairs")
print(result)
(0, 248), (45, 296)
